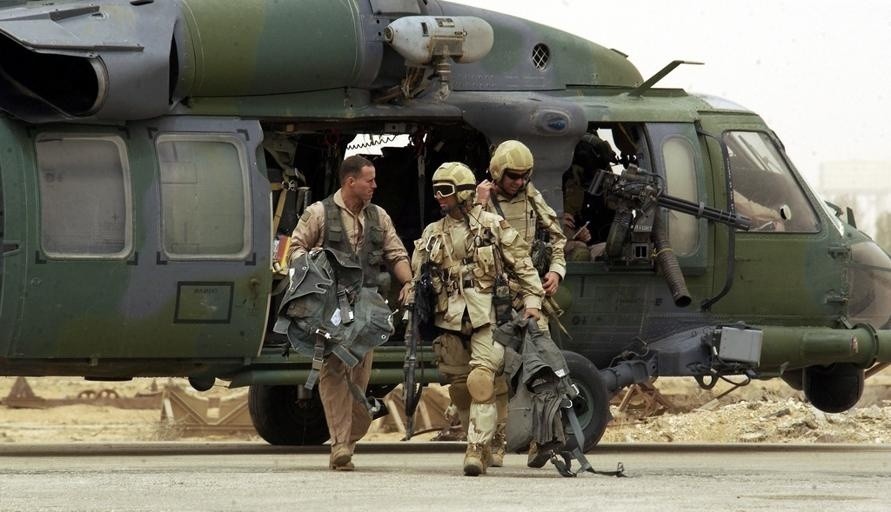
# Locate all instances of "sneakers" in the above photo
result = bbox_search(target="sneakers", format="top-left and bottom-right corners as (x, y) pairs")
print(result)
(329, 444), (354, 470)
(464, 439), (504, 476)
(527, 438), (552, 467)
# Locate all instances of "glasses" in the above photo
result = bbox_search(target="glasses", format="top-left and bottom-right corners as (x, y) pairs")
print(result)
(506, 172), (529, 180)
(433, 183), (456, 198)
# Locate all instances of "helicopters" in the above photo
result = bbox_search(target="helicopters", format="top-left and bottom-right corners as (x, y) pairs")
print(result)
(0, 0), (891, 455)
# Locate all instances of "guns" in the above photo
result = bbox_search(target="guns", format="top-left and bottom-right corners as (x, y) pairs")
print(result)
(589, 164), (751, 255)
(403, 280), (432, 440)
(527, 217), (553, 278)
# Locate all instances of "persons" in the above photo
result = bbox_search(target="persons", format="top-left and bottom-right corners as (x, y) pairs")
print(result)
(284, 155), (414, 473)
(474, 139), (566, 469)
(736, 189), (787, 234)
(407, 160), (547, 476)
(561, 211), (591, 265)
(572, 132), (617, 241)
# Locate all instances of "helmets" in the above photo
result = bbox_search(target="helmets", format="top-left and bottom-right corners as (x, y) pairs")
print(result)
(489, 140), (533, 181)
(432, 162), (476, 203)
